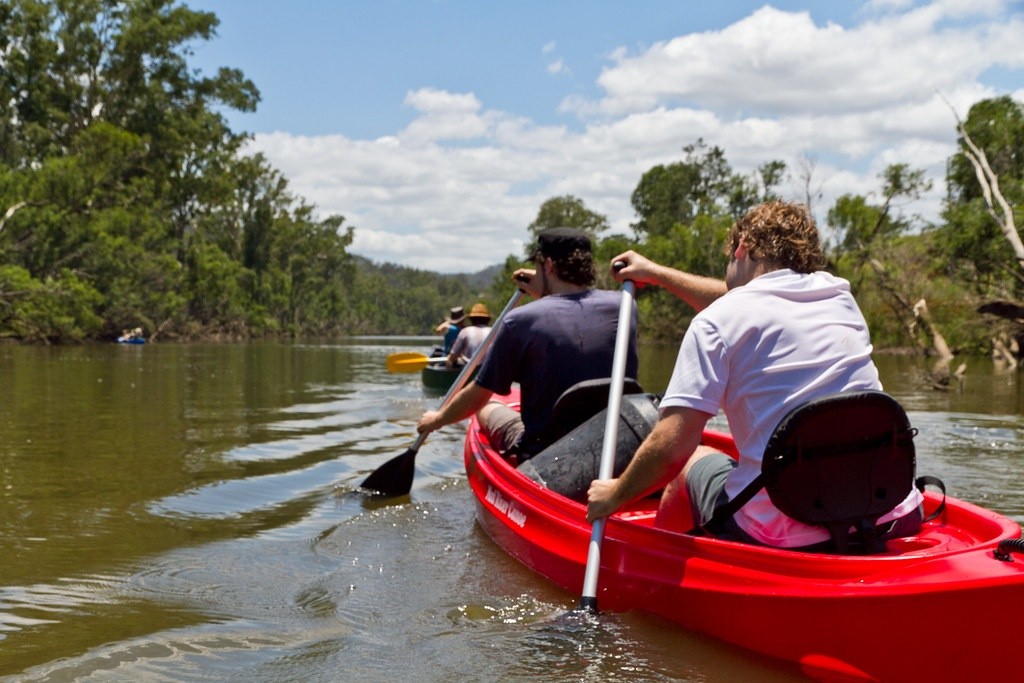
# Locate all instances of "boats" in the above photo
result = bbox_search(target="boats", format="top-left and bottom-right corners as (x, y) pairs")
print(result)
(422, 345), (474, 391)
(464, 385), (1024, 682)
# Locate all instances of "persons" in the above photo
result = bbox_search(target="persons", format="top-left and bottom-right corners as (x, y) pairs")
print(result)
(435, 303), (498, 368)
(586, 200), (924, 553)
(416, 226), (639, 466)
(122, 327), (143, 341)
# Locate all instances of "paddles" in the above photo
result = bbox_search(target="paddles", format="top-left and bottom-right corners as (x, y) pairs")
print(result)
(385, 350), (453, 375)
(565, 260), (639, 619)
(358, 271), (532, 500)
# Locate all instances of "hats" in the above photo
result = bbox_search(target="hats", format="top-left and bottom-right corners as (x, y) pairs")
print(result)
(466, 302), (492, 317)
(446, 305), (467, 324)
(521, 226), (593, 263)
